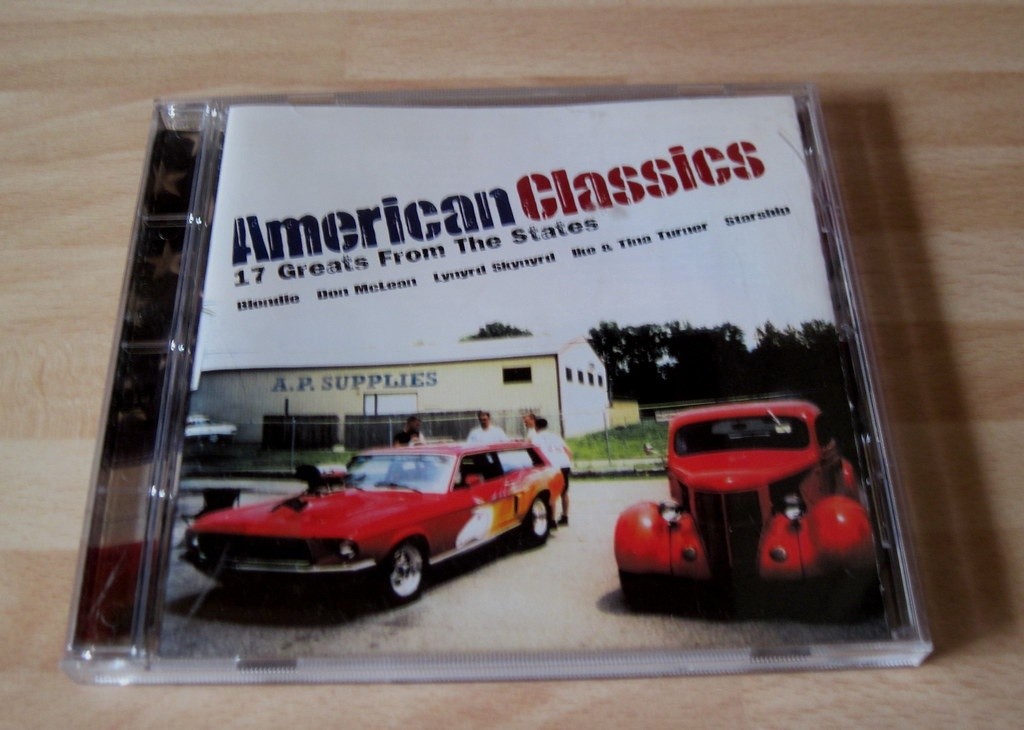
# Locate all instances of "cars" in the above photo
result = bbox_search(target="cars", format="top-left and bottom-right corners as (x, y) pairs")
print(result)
(613, 401), (876, 624)
(183, 438), (565, 606)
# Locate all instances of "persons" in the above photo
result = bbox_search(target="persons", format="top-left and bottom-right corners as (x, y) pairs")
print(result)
(392, 416), (427, 448)
(531, 419), (574, 526)
(522, 414), (536, 439)
(466, 411), (507, 443)
